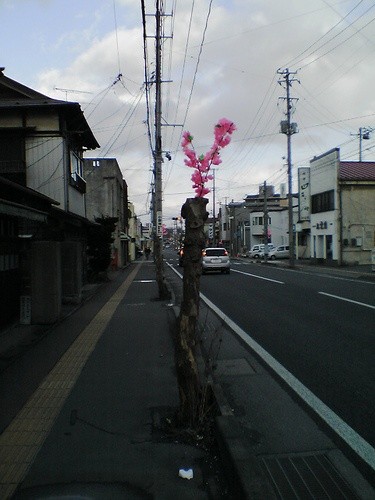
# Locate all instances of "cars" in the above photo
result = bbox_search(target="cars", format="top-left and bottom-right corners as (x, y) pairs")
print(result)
(246, 243), (273, 260)
(178, 247), (184, 266)
(200, 247), (233, 274)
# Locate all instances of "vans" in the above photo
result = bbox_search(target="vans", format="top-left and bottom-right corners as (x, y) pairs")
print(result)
(268, 245), (290, 260)
